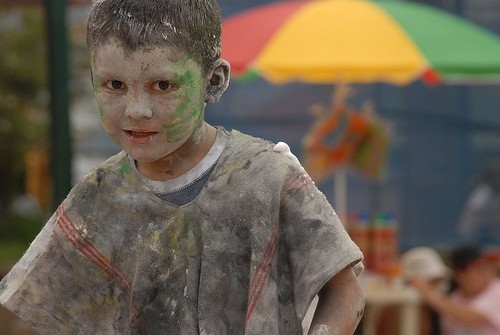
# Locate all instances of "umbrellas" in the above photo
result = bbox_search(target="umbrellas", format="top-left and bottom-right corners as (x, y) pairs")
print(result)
(217, 0), (500, 233)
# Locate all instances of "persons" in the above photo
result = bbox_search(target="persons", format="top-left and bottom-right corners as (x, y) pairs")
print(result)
(409, 242), (500, 334)
(0, 1), (369, 334)
